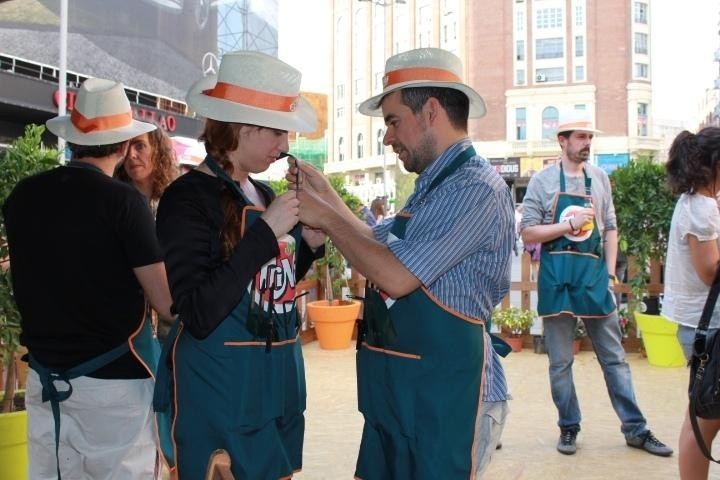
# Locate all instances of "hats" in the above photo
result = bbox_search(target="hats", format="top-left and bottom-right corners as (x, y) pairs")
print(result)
(45, 78), (158, 146)
(185, 51), (318, 133)
(360, 48), (486, 120)
(557, 110), (603, 135)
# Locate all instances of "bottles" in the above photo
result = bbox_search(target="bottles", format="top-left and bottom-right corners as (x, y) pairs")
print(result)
(583, 196), (595, 232)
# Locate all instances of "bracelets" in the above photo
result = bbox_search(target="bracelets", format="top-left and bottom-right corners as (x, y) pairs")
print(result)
(570, 218), (576, 231)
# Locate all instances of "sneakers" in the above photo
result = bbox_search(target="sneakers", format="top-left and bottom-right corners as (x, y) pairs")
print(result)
(627, 432), (673, 456)
(557, 432), (577, 454)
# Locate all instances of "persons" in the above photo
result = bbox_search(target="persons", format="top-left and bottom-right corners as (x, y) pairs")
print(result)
(1, 76), (180, 480)
(288, 46), (512, 480)
(155, 52), (327, 480)
(659, 126), (720, 480)
(518, 113), (676, 455)
(113, 123), (181, 480)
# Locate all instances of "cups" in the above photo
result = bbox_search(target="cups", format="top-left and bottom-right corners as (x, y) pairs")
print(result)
(639, 296), (659, 314)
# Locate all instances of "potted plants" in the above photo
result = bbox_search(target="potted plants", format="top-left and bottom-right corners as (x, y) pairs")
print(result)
(491, 308), (536, 351)
(573, 322), (585, 354)
(608, 152), (690, 369)
(266, 171), (362, 352)
(1, 123), (70, 478)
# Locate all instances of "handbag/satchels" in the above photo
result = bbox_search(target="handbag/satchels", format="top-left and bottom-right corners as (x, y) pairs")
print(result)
(690, 330), (720, 419)
